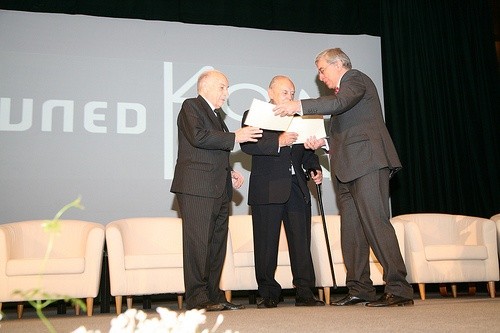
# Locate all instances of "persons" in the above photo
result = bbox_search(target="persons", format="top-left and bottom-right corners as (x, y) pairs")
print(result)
(273, 47), (414, 307)
(240, 75), (323, 308)
(169, 70), (262, 312)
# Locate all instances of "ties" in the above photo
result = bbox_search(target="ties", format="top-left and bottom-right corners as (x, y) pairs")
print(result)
(215, 109), (221, 117)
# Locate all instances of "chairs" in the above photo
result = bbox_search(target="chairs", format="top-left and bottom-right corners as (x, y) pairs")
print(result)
(0, 219), (105, 317)
(105, 218), (185, 314)
(218, 213), (326, 304)
(311, 215), (405, 305)
(396, 214), (500, 299)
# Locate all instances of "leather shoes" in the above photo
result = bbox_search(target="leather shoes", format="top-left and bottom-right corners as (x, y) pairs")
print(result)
(294, 297), (325, 307)
(331, 295), (367, 306)
(202, 304), (224, 312)
(365, 293), (414, 308)
(256, 298), (278, 309)
(220, 301), (245, 311)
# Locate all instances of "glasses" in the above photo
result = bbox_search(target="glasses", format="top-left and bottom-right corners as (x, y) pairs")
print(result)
(318, 62), (334, 74)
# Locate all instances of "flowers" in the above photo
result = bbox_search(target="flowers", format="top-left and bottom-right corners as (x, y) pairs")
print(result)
(15, 198), (238, 333)
